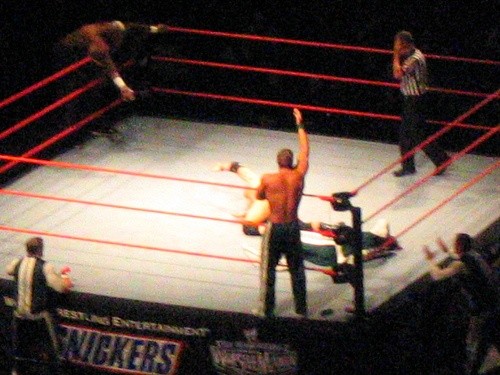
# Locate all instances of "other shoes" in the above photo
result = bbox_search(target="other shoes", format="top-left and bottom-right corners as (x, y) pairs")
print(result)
(435, 157), (456, 177)
(392, 168), (417, 177)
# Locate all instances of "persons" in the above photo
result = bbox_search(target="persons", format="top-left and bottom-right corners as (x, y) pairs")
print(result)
(7, 238), (74, 375)
(392, 30), (452, 175)
(209, 162), (345, 238)
(49, 21), (167, 149)
(256, 107), (310, 322)
(423, 233), (500, 375)
